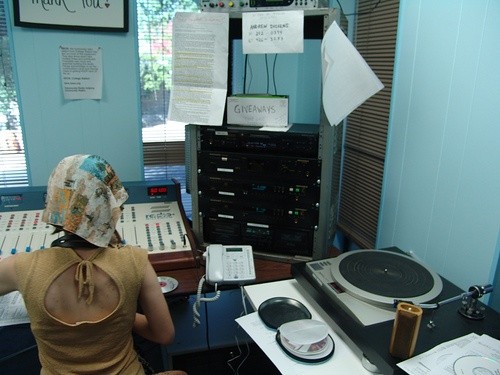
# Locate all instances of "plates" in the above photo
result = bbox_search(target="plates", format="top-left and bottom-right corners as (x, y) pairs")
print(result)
(158, 276), (178, 293)
(258, 297), (312, 330)
(275, 330), (335, 363)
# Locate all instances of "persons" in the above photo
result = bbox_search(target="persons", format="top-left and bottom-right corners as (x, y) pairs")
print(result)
(0, 155), (186, 375)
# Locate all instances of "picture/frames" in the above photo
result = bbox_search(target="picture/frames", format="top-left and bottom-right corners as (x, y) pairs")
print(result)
(13, 0), (129, 32)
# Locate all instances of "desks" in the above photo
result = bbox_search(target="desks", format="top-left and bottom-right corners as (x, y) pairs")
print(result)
(1, 253), (378, 375)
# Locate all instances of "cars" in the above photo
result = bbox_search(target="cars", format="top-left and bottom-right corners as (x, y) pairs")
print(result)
(0, 100), (23, 153)
(141, 109), (188, 143)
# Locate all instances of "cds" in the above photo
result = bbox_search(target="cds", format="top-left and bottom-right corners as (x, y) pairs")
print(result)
(156, 276), (179, 293)
(281, 333), (329, 355)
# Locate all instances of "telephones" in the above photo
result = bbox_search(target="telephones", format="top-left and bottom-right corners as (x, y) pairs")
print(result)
(202, 244), (256, 285)
